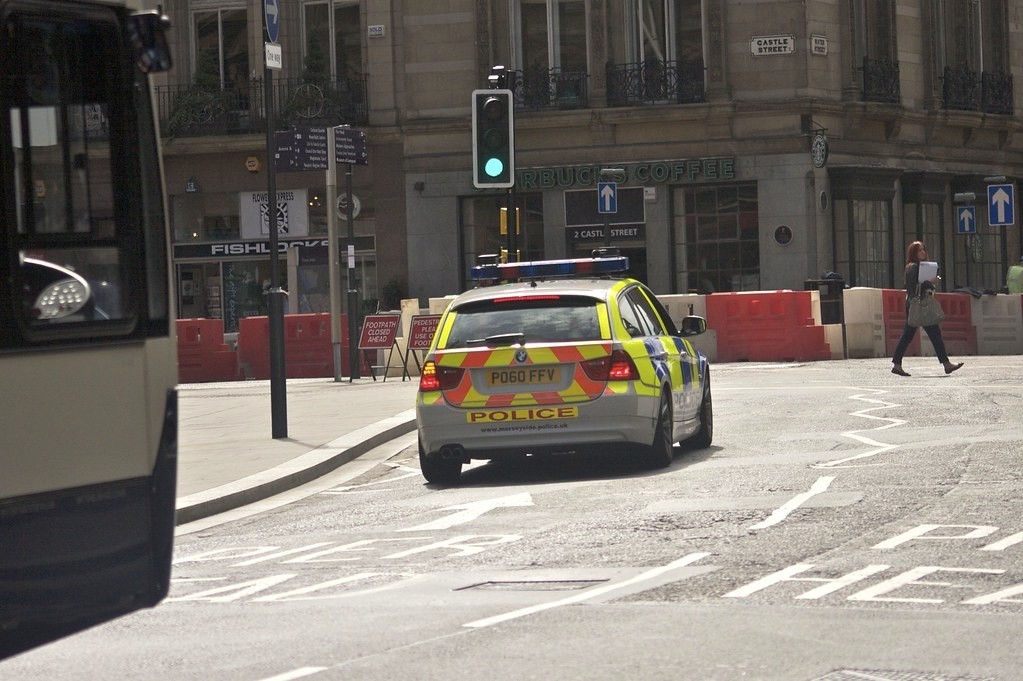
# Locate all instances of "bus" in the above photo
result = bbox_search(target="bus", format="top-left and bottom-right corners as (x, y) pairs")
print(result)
(0, 2), (187, 668)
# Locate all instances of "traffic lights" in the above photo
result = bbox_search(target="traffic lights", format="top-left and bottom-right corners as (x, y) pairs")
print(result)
(471, 89), (517, 189)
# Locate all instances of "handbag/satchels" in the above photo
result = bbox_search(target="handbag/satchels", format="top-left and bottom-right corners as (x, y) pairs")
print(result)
(908, 296), (944, 327)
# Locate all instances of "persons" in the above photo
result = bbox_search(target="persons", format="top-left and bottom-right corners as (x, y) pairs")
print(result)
(1005, 257), (1023, 293)
(892, 241), (965, 376)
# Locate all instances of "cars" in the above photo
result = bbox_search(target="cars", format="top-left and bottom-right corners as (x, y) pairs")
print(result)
(412, 253), (714, 485)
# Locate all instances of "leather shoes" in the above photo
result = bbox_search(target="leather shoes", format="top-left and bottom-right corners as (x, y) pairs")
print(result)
(945, 362), (964, 374)
(892, 368), (911, 376)
